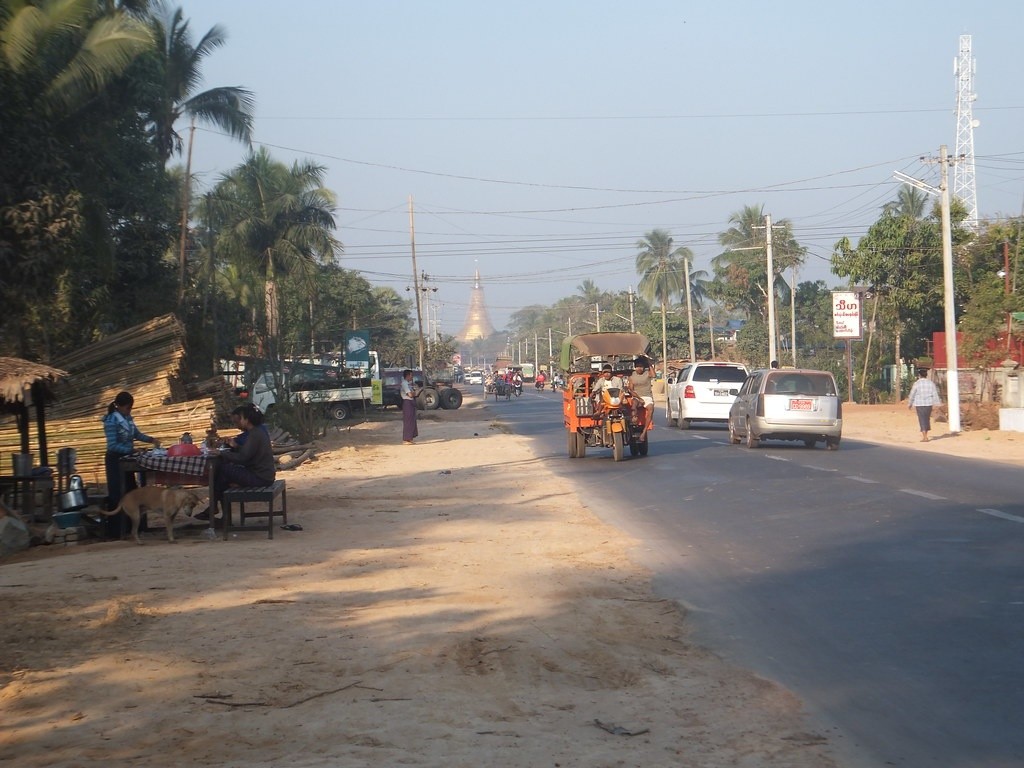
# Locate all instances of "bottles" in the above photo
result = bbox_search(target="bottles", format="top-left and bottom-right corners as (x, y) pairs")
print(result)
(181, 432), (192, 444)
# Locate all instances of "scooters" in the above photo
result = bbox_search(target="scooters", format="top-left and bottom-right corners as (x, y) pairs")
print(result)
(514, 382), (521, 397)
(536, 381), (544, 391)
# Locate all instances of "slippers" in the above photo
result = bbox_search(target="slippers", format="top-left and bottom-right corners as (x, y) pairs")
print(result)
(195, 512), (218, 520)
(280, 524), (303, 530)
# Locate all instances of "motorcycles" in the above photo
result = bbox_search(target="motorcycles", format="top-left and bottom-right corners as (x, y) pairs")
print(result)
(562, 332), (654, 458)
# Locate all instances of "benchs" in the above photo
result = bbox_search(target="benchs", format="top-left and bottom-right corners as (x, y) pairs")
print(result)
(221, 480), (288, 541)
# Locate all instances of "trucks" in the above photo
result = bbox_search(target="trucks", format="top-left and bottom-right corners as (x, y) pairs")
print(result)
(522, 364), (534, 381)
(251, 372), (373, 421)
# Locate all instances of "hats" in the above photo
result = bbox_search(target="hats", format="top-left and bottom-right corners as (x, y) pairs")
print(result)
(602, 364), (612, 373)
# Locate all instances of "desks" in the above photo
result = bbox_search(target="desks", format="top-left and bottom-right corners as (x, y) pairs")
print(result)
(117, 448), (231, 540)
(0, 475), (56, 522)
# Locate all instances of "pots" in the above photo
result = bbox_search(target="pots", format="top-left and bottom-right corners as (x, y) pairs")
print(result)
(54, 487), (87, 512)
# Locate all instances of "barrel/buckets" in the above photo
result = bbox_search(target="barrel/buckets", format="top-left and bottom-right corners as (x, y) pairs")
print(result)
(11, 452), (35, 476)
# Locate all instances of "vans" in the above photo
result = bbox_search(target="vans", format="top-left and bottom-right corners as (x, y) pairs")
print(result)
(470, 371), (483, 385)
(382, 368), (428, 410)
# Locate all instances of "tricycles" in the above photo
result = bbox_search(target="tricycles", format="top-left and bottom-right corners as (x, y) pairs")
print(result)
(485, 378), (510, 403)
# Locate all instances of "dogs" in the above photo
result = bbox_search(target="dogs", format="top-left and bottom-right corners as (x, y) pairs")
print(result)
(90, 486), (205, 545)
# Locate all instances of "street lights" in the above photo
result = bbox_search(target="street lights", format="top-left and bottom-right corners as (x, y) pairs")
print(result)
(407, 286), (426, 346)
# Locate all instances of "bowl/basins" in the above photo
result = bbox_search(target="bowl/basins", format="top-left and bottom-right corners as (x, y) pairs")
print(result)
(52, 511), (81, 529)
(167, 444), (201, 456)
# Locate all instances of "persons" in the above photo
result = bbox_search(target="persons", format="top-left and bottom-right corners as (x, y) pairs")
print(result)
(485, 369), (523, 399)
(771, 361), (778, 369)
(536, 370), (546, 389)
(627, 358), (656, 442)
(192, 404), (275, 529)
(554, 373), (563, 386)
(102, 392), (161, 537)
(400, 370), (423, 444)
(590, 365), (628, 447)
(908, 370), (939, 442)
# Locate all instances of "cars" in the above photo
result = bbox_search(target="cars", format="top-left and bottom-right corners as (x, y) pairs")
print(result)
(463, 374), (471, 384)
(729, 368), (841, 450)
(667, 363), (749, 429)
